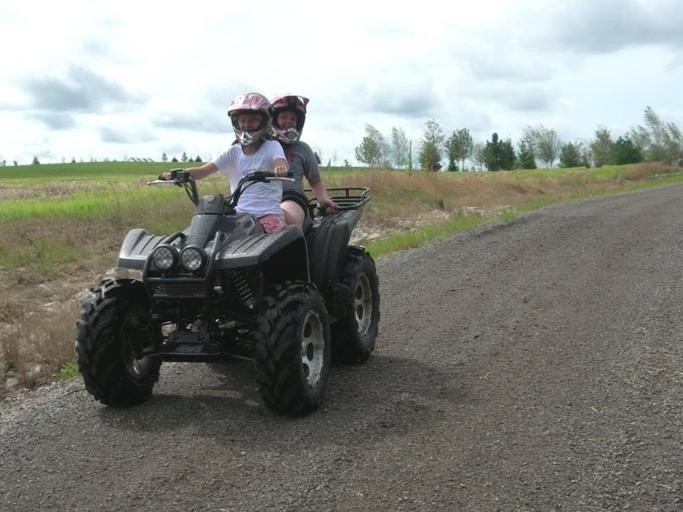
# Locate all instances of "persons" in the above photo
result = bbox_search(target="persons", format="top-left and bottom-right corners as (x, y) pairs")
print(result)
(264, 93), (340, 231)
(158, 91), (289, 233)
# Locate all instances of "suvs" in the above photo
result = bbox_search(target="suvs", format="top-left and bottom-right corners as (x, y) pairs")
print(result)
(75, 168), (380, 416)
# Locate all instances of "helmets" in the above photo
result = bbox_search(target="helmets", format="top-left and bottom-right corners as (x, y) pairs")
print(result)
(270, 94), (310, 145)
(226, 92), (273, 146)
